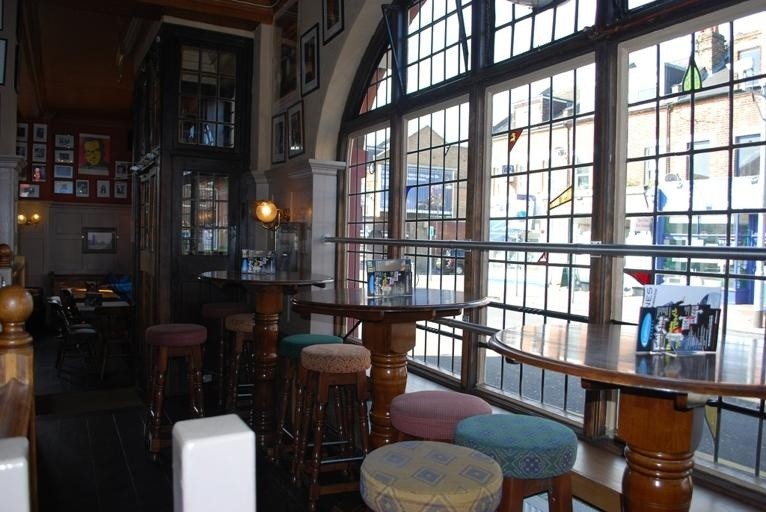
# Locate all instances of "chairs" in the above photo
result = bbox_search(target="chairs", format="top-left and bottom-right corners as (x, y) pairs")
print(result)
(27, 271), (141, 385)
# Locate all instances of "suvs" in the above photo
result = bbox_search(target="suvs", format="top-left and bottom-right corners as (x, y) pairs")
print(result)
(434, 244), (465, 276)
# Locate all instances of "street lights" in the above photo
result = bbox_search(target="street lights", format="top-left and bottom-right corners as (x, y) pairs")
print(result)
(561, 102), (576, 286)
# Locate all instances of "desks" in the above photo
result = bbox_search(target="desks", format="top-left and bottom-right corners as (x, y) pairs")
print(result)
(290, 288), (490, 451)
(199, 268), (334, 461)
(489, 323), (766, 512)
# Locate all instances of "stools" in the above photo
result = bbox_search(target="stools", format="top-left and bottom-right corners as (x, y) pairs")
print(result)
(275, 334), (343, 488)
(360, 441), (503, 512)
(454, 414), (578, 512)
(391, 391), (492, 442)
(295, 344), (371, 512)
(203, 303), (256, 415)
(144, 323), (206, 464)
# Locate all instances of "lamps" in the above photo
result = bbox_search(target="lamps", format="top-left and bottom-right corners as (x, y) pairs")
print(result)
(16, 209), (43, 227)
(246, 196), (293, 233)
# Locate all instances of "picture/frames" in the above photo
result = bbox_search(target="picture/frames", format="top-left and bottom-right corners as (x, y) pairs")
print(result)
(271, 0), (347, 169)
(13, 120), (132, 202)
(0, 37), (8, 86)
(0, 0), (5, 32)
(79, 225), (119, 254)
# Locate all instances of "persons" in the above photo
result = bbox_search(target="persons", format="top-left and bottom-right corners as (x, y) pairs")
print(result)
(18, 148), (24, 154)
(33, 167), (42, 180)
(118, 166), (125, 176)
(81, 139), (110, 170)
(289, 115), (299, 148)
